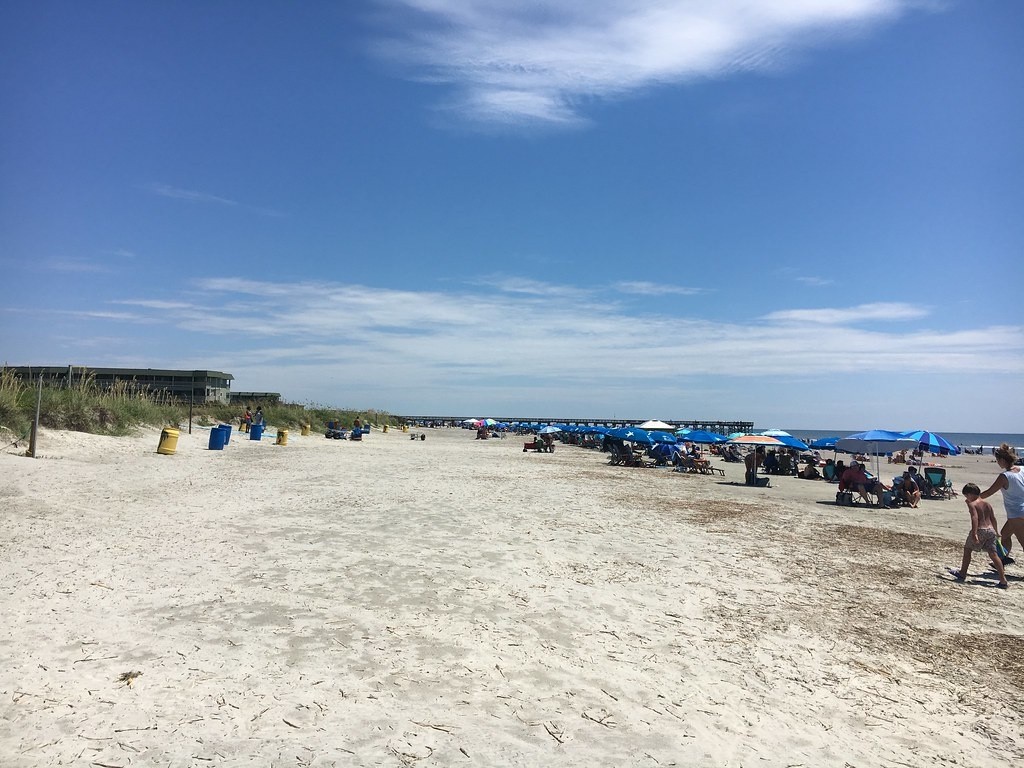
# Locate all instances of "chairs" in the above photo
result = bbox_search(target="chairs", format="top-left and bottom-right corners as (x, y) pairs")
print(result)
(672, 452), (726, 477)
(607, 441), (656, 468)
(924, 467), (954, 500)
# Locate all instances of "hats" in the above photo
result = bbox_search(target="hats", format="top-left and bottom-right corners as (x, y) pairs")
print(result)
(850, 461), (859, 466)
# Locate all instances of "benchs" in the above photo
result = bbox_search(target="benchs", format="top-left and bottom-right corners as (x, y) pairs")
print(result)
(523, 442), (547, 453)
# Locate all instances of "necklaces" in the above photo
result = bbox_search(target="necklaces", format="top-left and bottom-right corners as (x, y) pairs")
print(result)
(1007, 467), (1016, 471)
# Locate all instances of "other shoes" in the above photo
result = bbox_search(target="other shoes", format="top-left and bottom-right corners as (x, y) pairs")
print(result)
(989, 563), (997, 569)
(881, 504), (890, 509)
(246, 431), (248, 433)
(865, 504), (873, 508)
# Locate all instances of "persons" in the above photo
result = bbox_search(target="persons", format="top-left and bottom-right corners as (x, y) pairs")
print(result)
(561, 434), (710, 475)
(957, 444), (998, 455)
(244, 405), (263, 433)
(540, 433), (555, 453)
(838, 461), (936, 509)
(965, 443), (1024, 571)
(476, 426), (499, 440)
(709, 444), (742, 463)
(753, 446), (838, 481)
(948, 482), (1008, 589)
(354, 416), (359, 428)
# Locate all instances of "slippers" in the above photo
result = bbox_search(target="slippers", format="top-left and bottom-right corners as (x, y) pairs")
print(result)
(995, 582), (1007, 588)
(949, 570), (965, 580)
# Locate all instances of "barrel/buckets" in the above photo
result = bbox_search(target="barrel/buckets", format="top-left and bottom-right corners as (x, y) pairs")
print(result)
(209, 428), (226, 450)
(365, 425), (370, 430)
(219, 424), (232, 445)
(157, 428), (179, 455)
(276, 429), (288, 444)
(301, 424), (311, 435)
(326, 421), (348, 431)
(251, 425), (261, 440)
(404, 426), (409, 433)
(383, 425), (389, 433)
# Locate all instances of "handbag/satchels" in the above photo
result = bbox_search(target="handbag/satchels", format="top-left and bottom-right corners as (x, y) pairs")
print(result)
(245, 412), (251, 419)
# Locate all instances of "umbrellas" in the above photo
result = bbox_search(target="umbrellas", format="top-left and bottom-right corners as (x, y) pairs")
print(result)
(463, 418), (622, 437)
(537, 426), (562, 433)
(726, 435), (786, 486)
(835, 429), (918, 505)
(614, 419), (729, 457)
(897, 430), (957, 477)
(726, 428), (850, 466)
